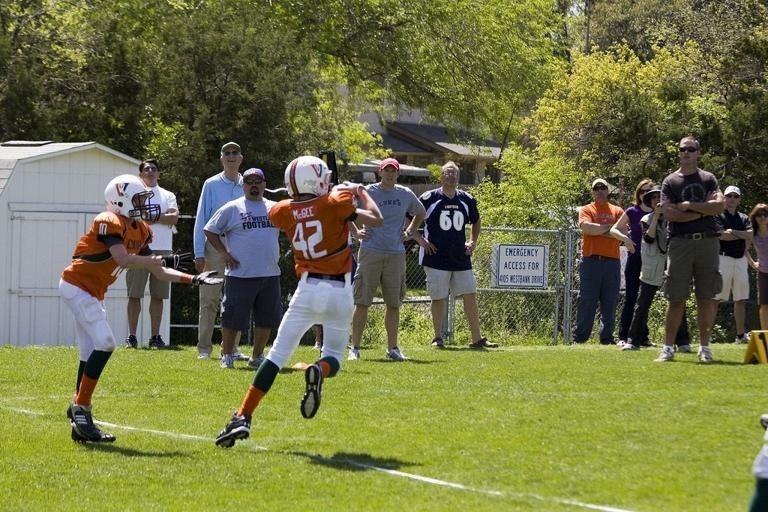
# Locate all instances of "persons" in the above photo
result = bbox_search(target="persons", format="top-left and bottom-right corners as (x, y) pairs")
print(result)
(58, 174), (223, 442)
(408, 162), (498, 347)
(347, 158), (426, 361)
(752, 414), (768, 510)
(193, 142), (253, 359)
(214, 156), (384, 451)
(711, 186), (767, 344)
(653, 137), (724, 363)
(126, 159), (179, 348)
(573, 177), (691, 353)
(203, 168), (282, 369)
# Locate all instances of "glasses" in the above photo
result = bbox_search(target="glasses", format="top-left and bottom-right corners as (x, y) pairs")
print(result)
(244, 178), (262, 184)
(592, 146), (766, 219)
(223, 151), (239, 156)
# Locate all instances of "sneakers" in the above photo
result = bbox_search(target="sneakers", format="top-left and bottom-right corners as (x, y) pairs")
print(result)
(570, 333), (751, 364)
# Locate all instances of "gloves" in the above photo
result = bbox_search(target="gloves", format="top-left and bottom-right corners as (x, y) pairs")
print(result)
(160, 249), (194, 272)
(337, 180), (365, 197)
(192, 270), (224, 286)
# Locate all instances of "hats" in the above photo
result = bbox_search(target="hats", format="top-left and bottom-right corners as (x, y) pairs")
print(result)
(381, 158), (399, 171)
(243, 168), (264, 180)
(221, 141), (241, 152)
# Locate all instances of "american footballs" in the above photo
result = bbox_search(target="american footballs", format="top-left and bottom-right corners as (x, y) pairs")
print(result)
(331, 187), (358, 207)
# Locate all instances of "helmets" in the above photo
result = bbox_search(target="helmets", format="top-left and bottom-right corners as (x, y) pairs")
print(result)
(284, 156), (334, 199)
(103, 173), (155, 223)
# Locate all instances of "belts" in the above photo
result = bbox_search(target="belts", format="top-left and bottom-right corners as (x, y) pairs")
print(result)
(308, 272), (345, 281)
(676, 231), (721, 241)
(589, 254), (615, 261)
